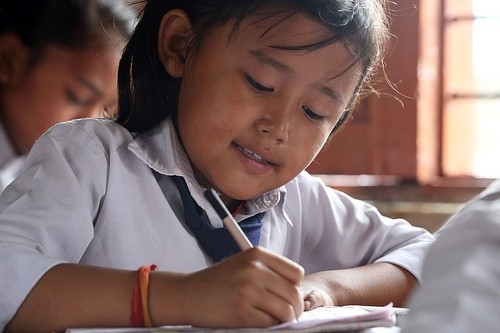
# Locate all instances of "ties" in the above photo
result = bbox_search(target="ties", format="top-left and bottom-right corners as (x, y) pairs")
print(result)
(173, 177), (265, 263)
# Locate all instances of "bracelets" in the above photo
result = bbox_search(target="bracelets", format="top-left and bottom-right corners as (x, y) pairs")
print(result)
(131, 264), (159, 328)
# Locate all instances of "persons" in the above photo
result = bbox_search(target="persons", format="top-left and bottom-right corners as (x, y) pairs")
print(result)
(0, 1), (435, 333)
(0, 1), (143, 193)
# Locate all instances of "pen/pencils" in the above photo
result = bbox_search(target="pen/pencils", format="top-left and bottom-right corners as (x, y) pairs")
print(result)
(203, 187), (299, 323)
